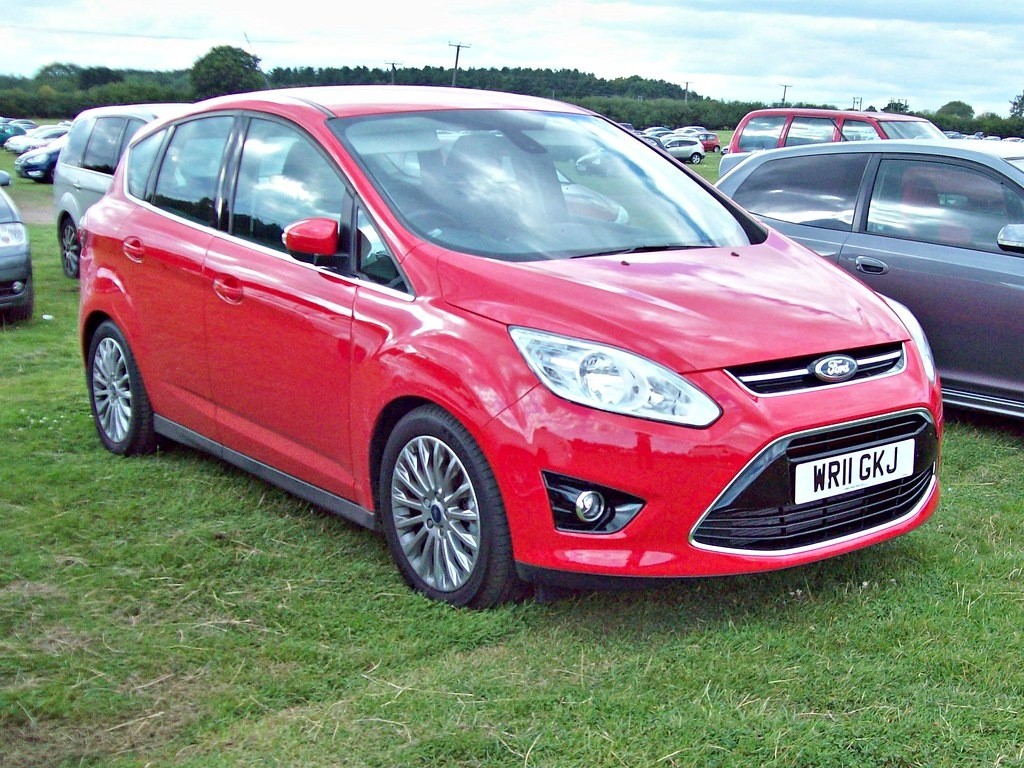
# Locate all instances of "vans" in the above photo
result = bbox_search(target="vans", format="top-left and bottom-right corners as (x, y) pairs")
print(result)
(723, 108), (1010, 252)
(53, 104), (237, 280)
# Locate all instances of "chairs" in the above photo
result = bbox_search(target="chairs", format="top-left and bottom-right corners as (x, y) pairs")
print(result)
(441, 134), (525, 241)
(897, 177), (972, 245)
(276, 140), (363, 232)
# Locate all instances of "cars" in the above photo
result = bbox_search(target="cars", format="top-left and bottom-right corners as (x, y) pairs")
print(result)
(78, 85), (945, 613)
(941, 130), (1024, 143)
(15, 132), (67, 184)
(710, 140), (1024, 423)
(0, 171), (35, 324)
(576, 122), (721, 178)
(0, 117), (75, 158)
